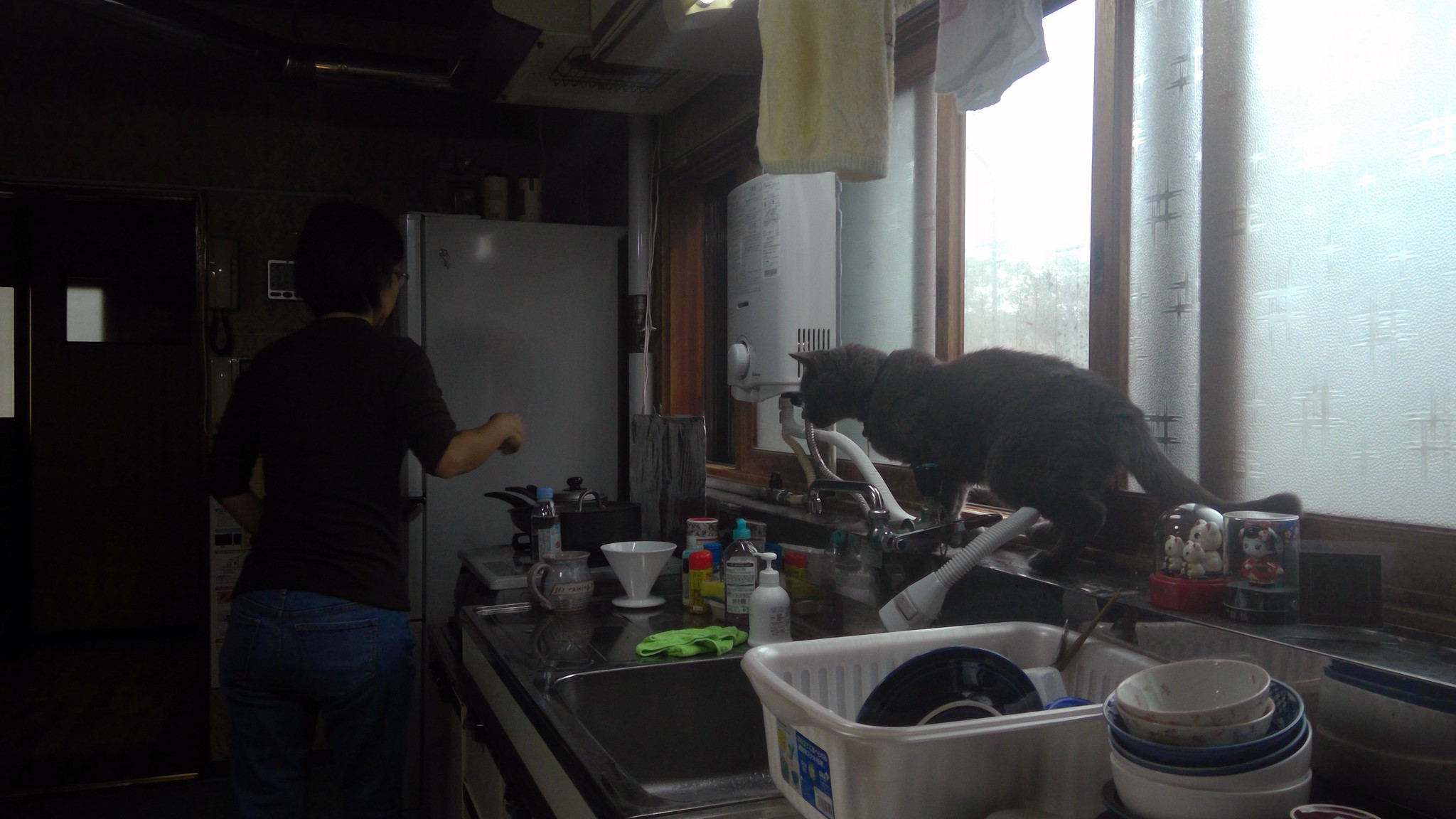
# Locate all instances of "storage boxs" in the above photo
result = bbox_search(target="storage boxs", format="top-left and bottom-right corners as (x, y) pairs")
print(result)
(736, 617), (1157, 819)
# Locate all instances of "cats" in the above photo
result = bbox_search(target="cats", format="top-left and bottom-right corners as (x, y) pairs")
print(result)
(790, 343), (1301, 573)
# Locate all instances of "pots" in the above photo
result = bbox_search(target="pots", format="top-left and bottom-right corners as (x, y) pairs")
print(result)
(485, 476), (642, 564)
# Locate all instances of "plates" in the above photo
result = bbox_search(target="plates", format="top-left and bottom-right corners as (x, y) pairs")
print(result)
(1289, 804), (1380, 819)
(853, 643), (1044, 729)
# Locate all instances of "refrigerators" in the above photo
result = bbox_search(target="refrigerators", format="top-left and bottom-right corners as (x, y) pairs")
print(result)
(400, 212), (619, 819)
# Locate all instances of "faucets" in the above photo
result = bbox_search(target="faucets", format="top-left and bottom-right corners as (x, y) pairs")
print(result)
(807, 479), (892, 552)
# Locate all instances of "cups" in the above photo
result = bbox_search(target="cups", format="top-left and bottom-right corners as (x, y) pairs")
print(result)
(484, 175), (541, 221)
(527, 550), (595, 611)
(600, 541), (677, 606)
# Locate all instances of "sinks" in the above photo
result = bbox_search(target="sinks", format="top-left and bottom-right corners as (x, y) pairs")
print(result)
(553, 655), (785, 819)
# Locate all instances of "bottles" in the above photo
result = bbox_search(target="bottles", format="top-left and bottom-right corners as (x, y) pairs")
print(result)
(681, 518), (766, 624)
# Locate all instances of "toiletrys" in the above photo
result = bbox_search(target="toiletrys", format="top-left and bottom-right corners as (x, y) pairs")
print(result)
(747, 552), (793, 646)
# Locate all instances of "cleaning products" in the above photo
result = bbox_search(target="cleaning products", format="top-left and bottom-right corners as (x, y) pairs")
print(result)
(725, 518), (760, 622)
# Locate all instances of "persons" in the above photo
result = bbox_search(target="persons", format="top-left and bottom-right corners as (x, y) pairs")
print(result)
(204, 200), (526, 819)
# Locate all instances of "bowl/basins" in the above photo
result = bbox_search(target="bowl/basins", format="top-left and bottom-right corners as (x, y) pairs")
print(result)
(1101, 658), (1314, 819)
